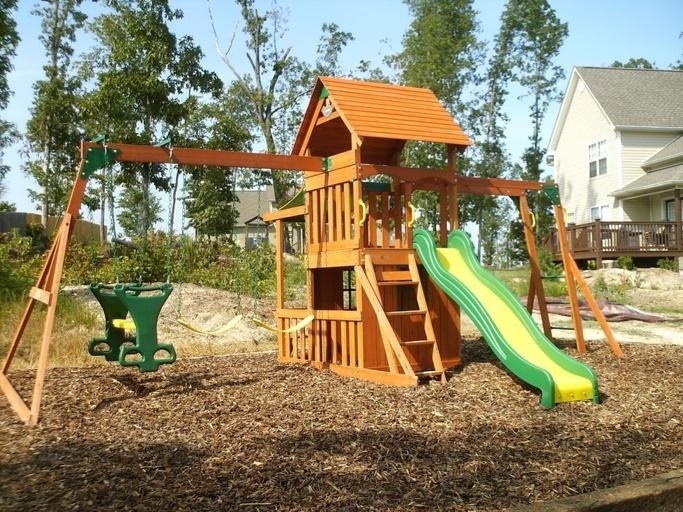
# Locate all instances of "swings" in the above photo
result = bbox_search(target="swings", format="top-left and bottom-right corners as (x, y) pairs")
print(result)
(88, 151), (178, 371)
(178, 164), (244, 336)
(253, 169), (317, 334)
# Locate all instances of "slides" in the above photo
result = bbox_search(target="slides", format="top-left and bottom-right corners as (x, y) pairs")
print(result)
(411, 228), (599, 409)
